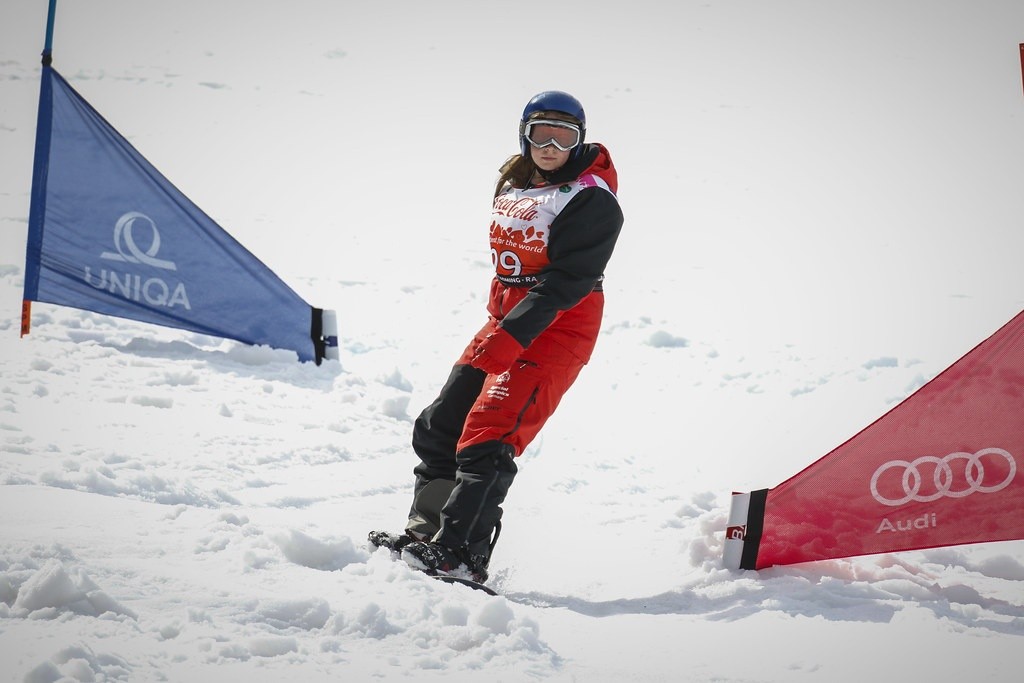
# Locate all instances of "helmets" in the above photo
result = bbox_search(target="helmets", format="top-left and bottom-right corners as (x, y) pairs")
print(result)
(519, 91), (586, 160)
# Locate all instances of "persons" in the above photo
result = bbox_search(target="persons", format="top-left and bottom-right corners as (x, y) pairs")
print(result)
(367, 92), (625, 584)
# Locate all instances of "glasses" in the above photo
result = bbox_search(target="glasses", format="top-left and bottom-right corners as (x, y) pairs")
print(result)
(524, 118), (580, 151)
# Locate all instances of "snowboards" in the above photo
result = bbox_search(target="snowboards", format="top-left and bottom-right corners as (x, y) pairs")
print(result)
(425, 573), (499, 597)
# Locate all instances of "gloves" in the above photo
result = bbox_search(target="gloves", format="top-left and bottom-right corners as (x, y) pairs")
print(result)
(471, 325), (525, 375)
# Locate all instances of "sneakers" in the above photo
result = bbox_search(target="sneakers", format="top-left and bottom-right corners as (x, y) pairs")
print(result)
(401, 541), (488, 584)
(368, 531), (414, 554)
